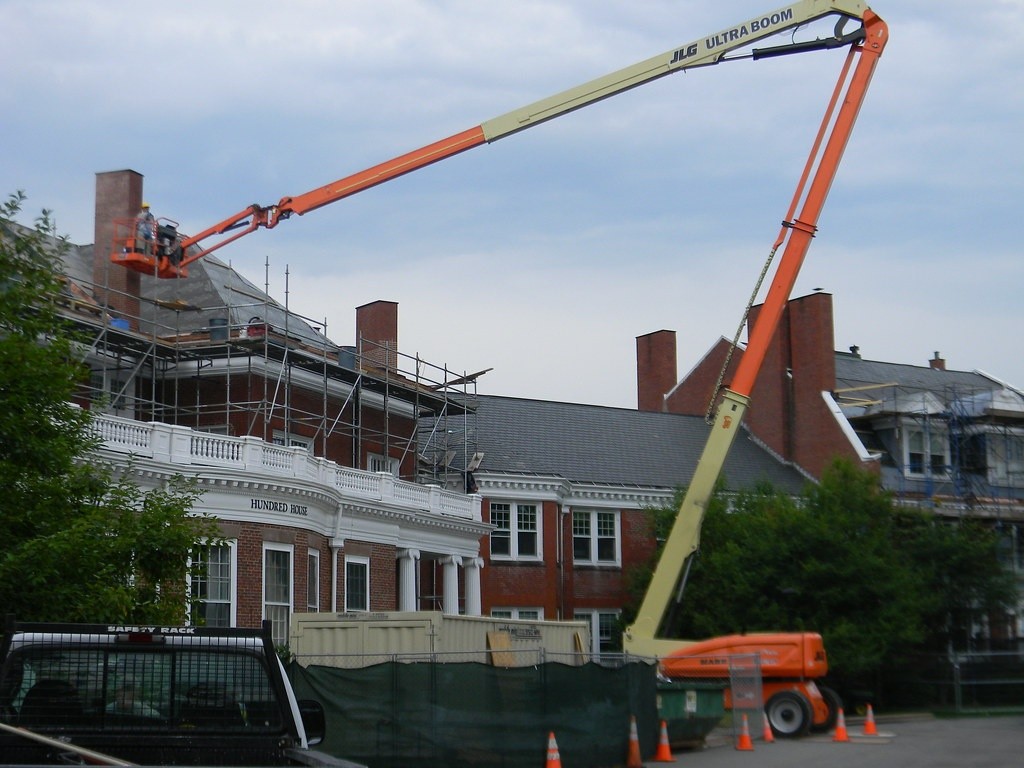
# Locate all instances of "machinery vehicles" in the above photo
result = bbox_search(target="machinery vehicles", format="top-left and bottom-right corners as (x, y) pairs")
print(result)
(107, 1), (867, 736)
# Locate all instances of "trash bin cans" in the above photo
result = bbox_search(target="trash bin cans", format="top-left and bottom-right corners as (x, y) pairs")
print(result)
(338, 345), (358, 371)
(209, 318), (229, 345)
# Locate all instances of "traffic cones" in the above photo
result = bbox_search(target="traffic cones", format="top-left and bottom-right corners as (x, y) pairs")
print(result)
(545, 731), (564, 768)
(651, 720), (678, 762)
(624, 715), (647, 768)
(863, 704), (878, 736)
(760, 710), (776, 743)
(735, 713), (755, 751)
(832, 707), (850, 742)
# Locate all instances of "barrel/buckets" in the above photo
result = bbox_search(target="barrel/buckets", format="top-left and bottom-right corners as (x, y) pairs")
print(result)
(208, 318), (229, 341)
(110, 319), (129, 330)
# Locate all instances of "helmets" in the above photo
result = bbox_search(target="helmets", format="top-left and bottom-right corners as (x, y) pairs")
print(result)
(141, 202), (150, 209)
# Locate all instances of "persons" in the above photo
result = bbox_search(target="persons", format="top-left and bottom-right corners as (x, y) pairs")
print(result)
(136, 203), (155, 255)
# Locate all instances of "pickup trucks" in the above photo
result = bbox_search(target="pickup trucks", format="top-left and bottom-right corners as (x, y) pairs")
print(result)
(0, 633), (381, 768)
